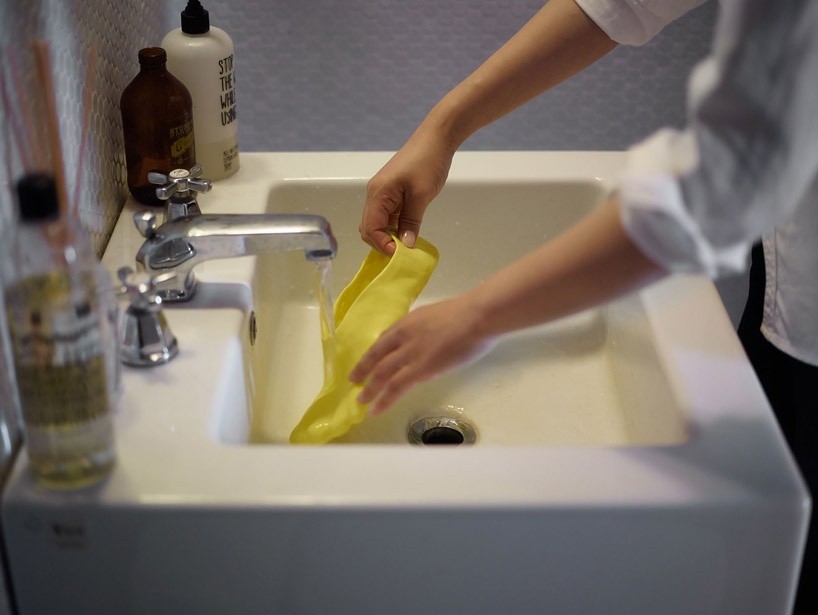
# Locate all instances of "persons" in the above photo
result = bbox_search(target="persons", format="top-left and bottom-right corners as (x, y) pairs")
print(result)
(347, 0), (818, 615)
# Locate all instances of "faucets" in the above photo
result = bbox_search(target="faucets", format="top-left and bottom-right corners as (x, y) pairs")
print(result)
(129, 208), (340, 308)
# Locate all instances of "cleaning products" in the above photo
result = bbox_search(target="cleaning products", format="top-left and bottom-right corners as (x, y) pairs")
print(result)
(117, 47), (200, 209)
(5, 167), (121, 493)
(159, 0), (242, 183)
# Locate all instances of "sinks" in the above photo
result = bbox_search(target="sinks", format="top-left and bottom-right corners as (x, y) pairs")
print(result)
(0, 145), (816, 614)
